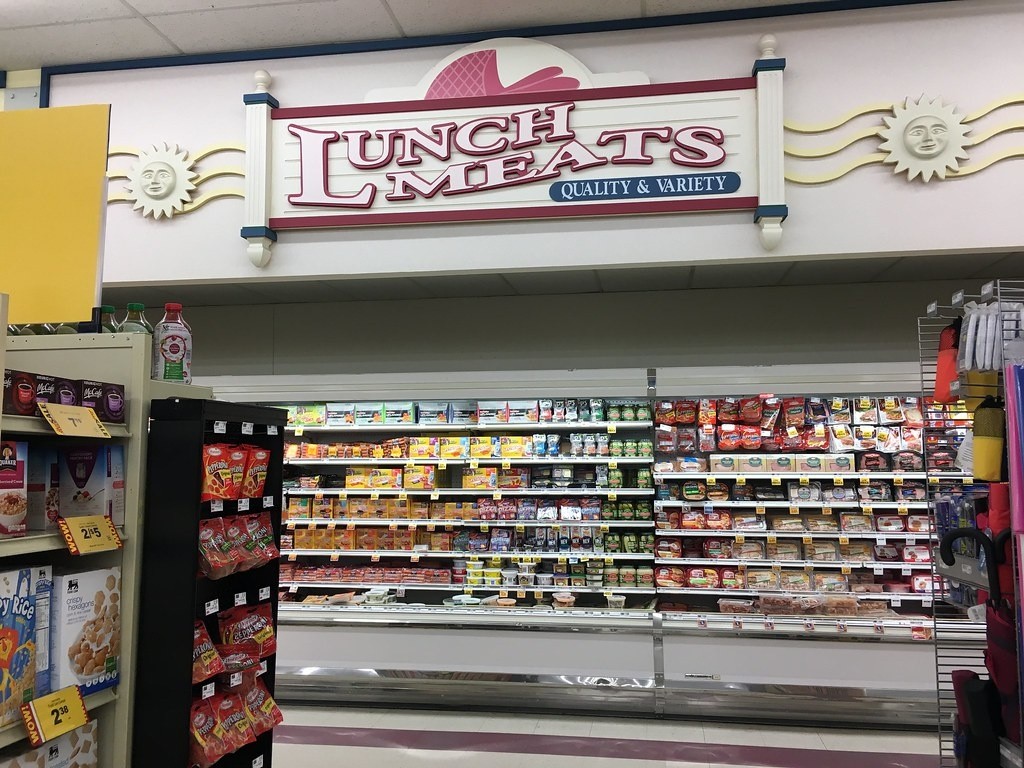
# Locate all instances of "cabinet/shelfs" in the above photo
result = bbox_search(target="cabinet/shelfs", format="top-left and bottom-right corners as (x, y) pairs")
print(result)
(0, 293), (977, 766)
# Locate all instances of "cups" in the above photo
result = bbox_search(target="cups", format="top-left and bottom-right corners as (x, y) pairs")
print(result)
(108, 394), (123, 411)
(17, 384), (34, 404)
(60, 390), (75, 405)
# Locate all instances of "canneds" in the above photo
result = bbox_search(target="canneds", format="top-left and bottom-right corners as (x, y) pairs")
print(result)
(602, 404), (654, 587)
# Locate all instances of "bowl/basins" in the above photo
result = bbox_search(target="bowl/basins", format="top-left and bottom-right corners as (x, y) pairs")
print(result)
(0, 491), (27, 529)
(67, 659), (107, 685)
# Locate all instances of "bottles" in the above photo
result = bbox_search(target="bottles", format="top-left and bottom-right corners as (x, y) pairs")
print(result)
(604, 565), (653, 587)
(605, 533), (655, 553)
(151, 303), (193, 385)
(7, 305), (120, 336)
(116, 303), (154, 332)
(607, 404), (652, 421)
(608, 468), (653, 488)
(602, 500), (652, 520)
(609, 438), (654, 457)
